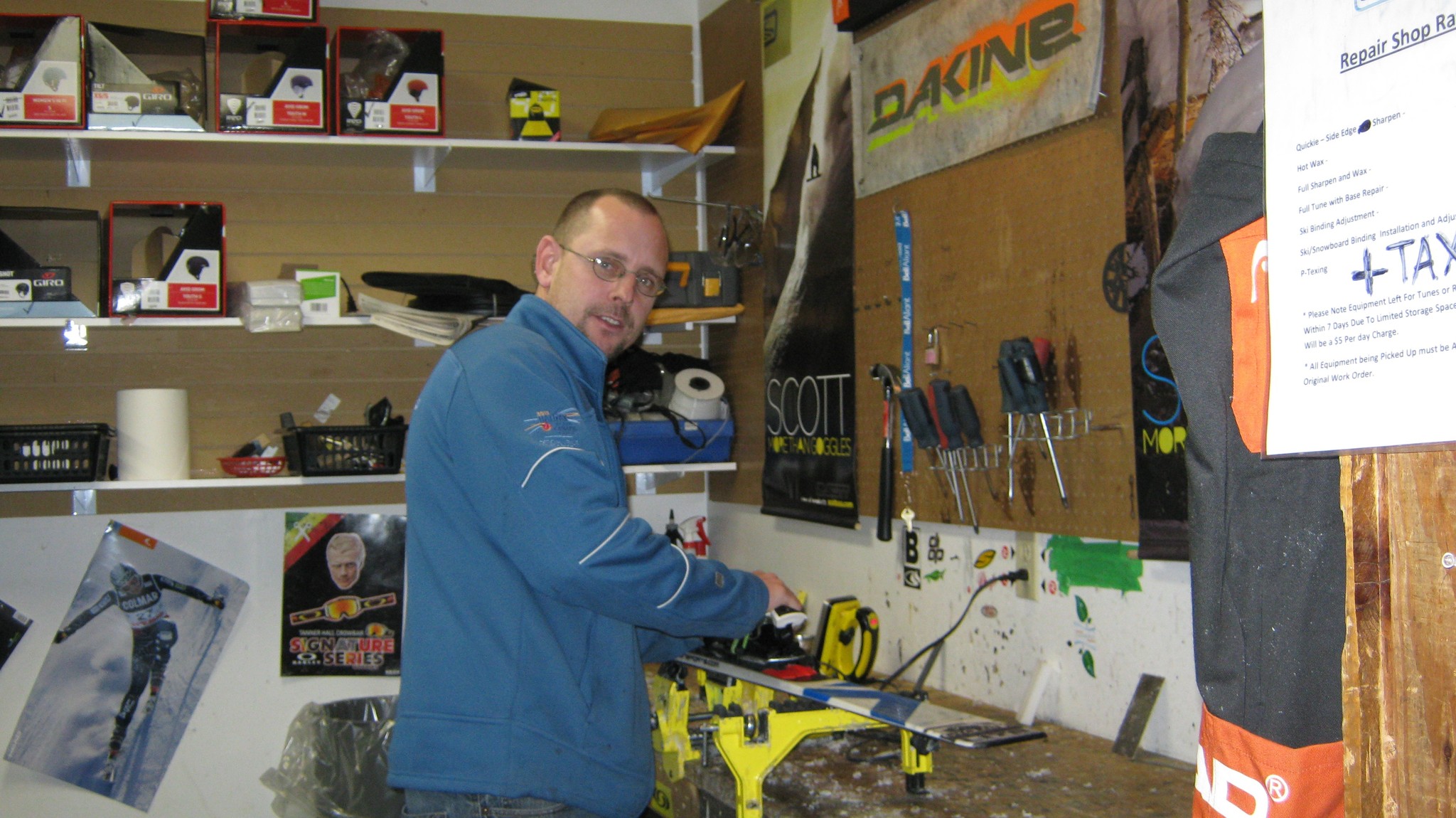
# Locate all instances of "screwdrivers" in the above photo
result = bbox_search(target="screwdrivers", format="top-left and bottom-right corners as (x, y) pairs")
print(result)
(897, 336), (1070, 537)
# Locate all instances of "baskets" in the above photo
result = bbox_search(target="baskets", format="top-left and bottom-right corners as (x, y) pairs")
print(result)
(280, 412), (404, 476)
(216, 457), (287, 477)
(0, 422), (119, 483)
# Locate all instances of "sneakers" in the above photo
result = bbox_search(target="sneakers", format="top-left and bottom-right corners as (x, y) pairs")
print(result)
(110, 729), (125, 750)
(150, 673), (164, 686)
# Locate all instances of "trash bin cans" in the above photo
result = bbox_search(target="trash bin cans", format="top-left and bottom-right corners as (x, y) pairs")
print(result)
(258, 693), (406, 818)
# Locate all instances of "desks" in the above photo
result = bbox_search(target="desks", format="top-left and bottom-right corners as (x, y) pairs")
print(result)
(642, 660), (1201, 818)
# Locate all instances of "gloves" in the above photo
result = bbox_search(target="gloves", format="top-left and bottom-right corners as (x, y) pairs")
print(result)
(55, 631), (67, 644)
(210, 597), (225, 610)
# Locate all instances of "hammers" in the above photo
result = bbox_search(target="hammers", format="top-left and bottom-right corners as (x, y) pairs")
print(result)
(867, 362), (904, 541)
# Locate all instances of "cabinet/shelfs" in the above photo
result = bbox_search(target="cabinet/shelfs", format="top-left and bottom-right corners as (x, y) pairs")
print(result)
(0, 121), (738, 498)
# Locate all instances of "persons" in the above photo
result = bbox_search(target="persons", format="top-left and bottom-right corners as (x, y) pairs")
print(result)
(386, 185), (803, 816)
(286, 532), (393, 630)
(53, 562), (226, 782)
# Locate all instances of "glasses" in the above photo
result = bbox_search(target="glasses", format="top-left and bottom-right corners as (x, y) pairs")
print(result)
(557, 244), (668, 298)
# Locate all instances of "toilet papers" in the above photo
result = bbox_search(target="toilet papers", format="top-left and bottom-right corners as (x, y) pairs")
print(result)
(110, 384), (200, 485)
(671, 365), (729, 427)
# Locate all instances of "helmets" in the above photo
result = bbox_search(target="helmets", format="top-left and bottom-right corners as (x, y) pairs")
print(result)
(110, 563), (144, 596)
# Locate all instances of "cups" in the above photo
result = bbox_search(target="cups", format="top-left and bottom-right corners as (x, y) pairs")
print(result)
(113, 388), (190, 482)
